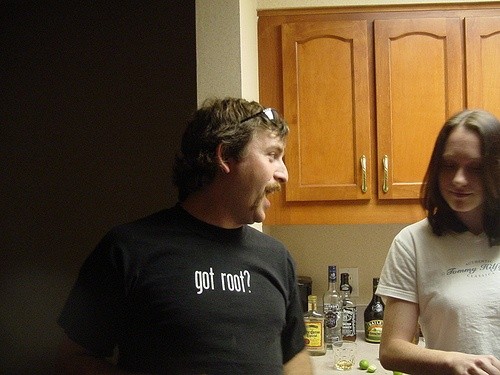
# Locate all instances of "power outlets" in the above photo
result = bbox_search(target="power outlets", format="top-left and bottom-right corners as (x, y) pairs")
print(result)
(338, 267), (359, 297)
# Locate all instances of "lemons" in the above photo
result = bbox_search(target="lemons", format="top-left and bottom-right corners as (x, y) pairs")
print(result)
(393, 371), (403, 375)
(368, 366), (377, 372)
(359, 360), (369, 369)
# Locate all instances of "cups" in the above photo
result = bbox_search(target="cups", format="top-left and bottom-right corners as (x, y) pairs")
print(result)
(332, 341), (357, 371)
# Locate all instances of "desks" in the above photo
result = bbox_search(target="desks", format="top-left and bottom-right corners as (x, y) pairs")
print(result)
(282, 328), (427, 375)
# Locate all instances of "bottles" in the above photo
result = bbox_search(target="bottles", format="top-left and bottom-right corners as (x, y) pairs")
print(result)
(303, 295), (326, 356)
(323, 266), (343, 349)
(364, 278), (385, 344)
(411, 322), (420, 345)
(337, 273), (357, 344)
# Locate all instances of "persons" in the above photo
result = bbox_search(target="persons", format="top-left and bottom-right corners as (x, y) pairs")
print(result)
(55, 96), (317, 375)
(374, 108), (500, 375)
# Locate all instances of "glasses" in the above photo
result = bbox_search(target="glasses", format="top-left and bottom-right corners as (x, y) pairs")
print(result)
(240, 108), (282, 127)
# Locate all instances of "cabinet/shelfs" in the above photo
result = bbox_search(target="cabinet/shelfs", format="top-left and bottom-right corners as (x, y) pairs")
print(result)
(256, 1), (500, 227)
